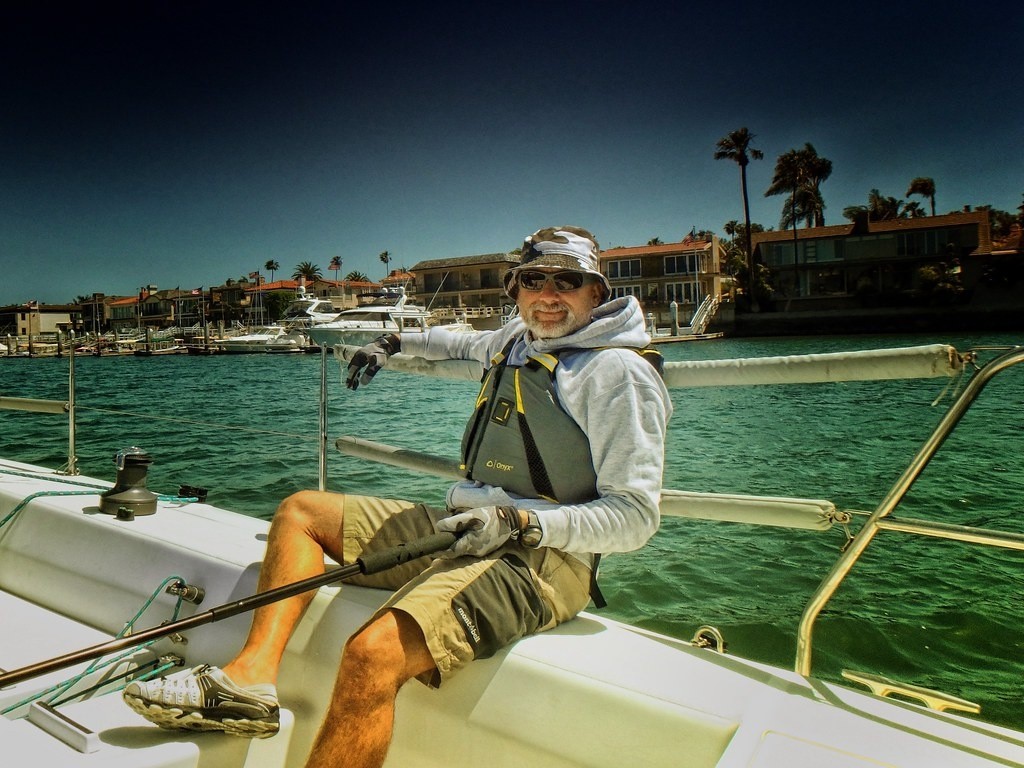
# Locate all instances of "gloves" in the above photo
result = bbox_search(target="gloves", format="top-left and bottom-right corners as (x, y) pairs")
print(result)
(345, 333), (400, 390)
(435, 506), (521, 558)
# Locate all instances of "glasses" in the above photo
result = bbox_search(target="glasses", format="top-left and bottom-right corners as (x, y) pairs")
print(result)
(517, 269), (593, 292)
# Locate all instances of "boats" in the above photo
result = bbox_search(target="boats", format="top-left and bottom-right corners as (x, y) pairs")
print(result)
(0, 264), (477, 357)
(0, 326), (1022, 766)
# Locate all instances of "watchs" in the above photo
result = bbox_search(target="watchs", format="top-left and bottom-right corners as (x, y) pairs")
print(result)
(519, 511), (543, 550)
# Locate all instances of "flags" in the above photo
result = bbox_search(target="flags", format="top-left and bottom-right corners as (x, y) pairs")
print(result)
(682, 229), (694, 246)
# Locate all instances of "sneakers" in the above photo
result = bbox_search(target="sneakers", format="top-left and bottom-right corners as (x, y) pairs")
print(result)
(122, 664), (280, 740)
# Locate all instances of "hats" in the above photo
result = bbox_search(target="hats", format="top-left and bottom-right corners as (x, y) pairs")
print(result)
(502, 225), (610, 306)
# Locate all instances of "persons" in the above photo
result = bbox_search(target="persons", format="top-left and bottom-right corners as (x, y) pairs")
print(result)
(126, 228), (675, 768)
